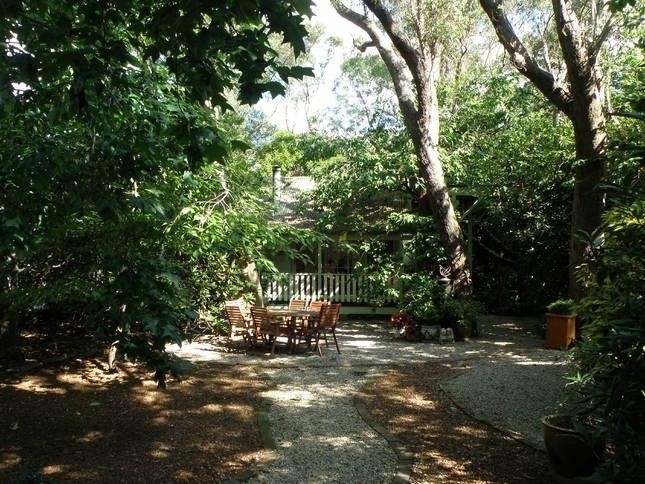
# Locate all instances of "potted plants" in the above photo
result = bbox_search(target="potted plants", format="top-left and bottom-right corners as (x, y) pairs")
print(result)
(546, 299), (578, 351)
(542, 365), (608, 478)
(391, 272), (484, 342)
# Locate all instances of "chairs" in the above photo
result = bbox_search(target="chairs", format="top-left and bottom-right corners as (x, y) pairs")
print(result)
(224, 300), (341, 356)
